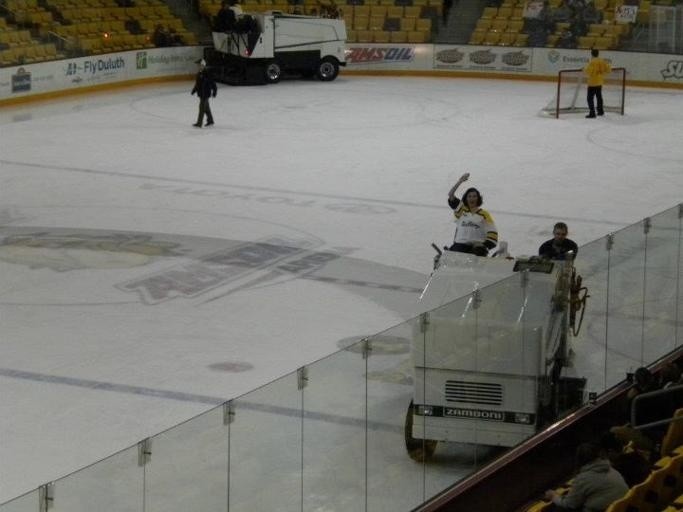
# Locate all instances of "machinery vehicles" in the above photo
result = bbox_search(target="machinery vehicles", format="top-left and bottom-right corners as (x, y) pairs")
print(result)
(404, 243), (586, 466)
(204, 3), (349, 87)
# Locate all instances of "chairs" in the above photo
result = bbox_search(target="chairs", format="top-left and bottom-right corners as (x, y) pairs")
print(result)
(468, 0), (672, 50)
(527, 442), (683, 512)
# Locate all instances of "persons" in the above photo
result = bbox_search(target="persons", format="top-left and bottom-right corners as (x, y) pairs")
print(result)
(169, 27), (189, 47)
(152, 24), (175, 47)
(539, 222), (578, 263)
(445, 171), (498, 257)
(519, 0), (603, 49)
(542, 360), (683, 512)
(214, 1), (235, 32)
(191, 58), (217, 128)
(583, 49), (611, 118)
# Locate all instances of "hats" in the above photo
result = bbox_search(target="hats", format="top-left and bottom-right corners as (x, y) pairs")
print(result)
(194, 58), (206, 66)
